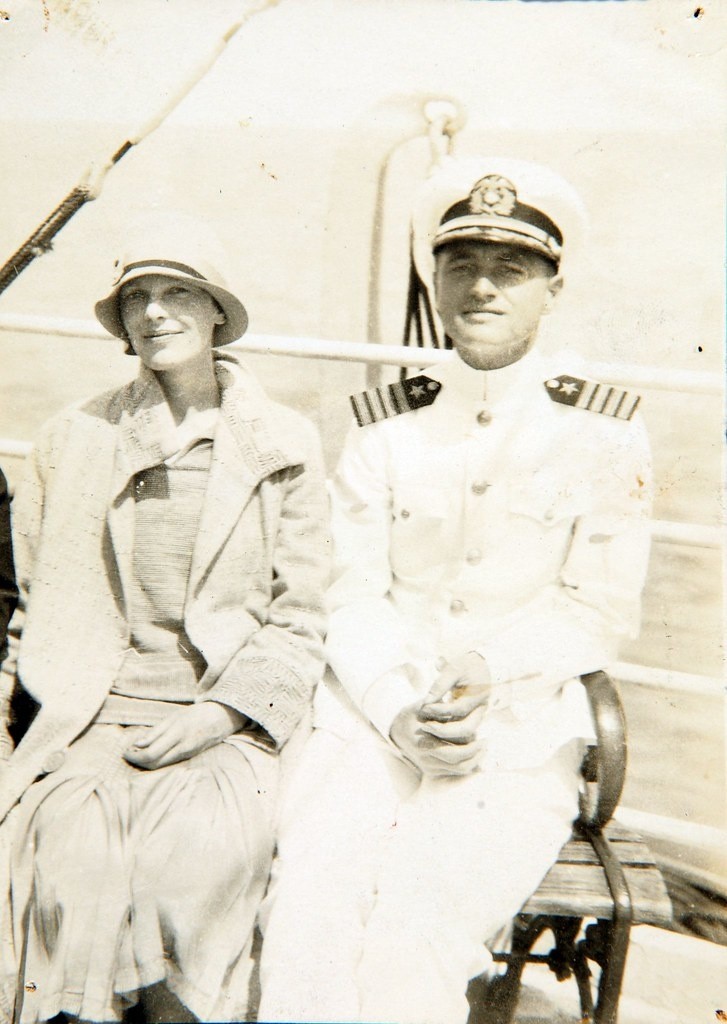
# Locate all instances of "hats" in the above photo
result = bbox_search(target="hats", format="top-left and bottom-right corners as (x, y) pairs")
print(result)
(96, 241), (249, 349)
(414, 159), (587, 284)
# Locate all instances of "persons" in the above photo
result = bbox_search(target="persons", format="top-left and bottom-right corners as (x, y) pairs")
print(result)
(255, 151), (659, 1024)
(0, 237), (334, 1023)
